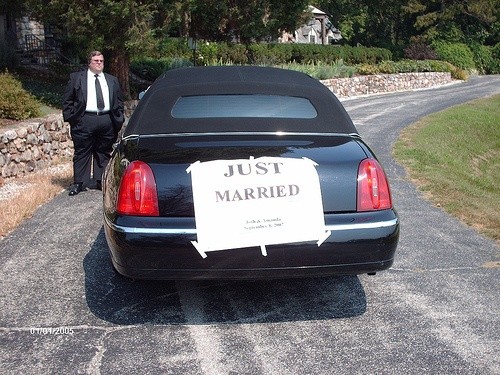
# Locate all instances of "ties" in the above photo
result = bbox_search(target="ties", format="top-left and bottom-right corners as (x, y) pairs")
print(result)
(94, 74), (105, 110)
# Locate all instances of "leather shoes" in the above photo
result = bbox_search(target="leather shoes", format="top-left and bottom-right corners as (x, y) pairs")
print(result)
(69, 182), (82, 196)
(96, 181), (101, 191)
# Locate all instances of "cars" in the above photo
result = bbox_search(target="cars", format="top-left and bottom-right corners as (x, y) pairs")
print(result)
(100, 65), (402, 281)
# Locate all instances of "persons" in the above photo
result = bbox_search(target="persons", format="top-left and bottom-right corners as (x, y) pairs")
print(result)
(63, 49), (123, 197)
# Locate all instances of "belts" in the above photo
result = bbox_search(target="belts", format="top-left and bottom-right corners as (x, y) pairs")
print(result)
(85, 111), (111, 116)
